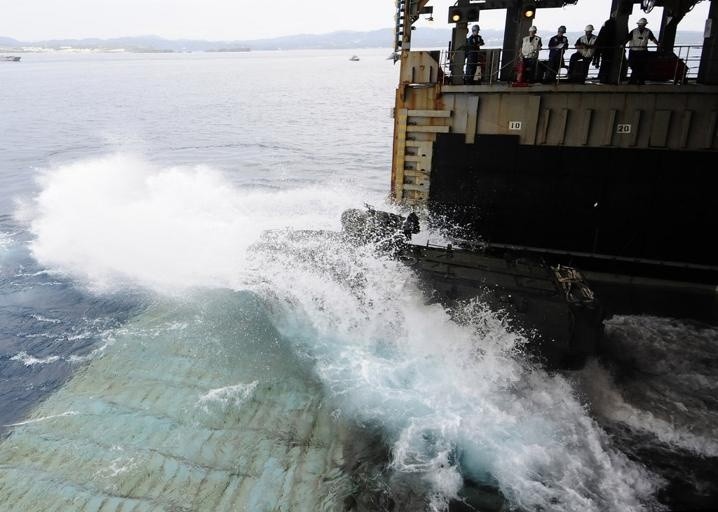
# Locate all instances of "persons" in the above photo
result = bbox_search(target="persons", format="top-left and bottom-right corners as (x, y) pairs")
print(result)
(519, 18), (661, 82)
(466, 25), (485, 80)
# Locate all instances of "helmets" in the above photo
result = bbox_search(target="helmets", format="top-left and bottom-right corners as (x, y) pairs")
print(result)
(636, 18), (648, 25)
(584, 24), (594, 31)
(558, 25), (566, 33)
(472, 24), (480, 31)
(528, 25), (537, 31)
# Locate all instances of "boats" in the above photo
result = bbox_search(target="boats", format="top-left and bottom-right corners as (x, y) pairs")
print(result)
(339, 0), (717, 319)
